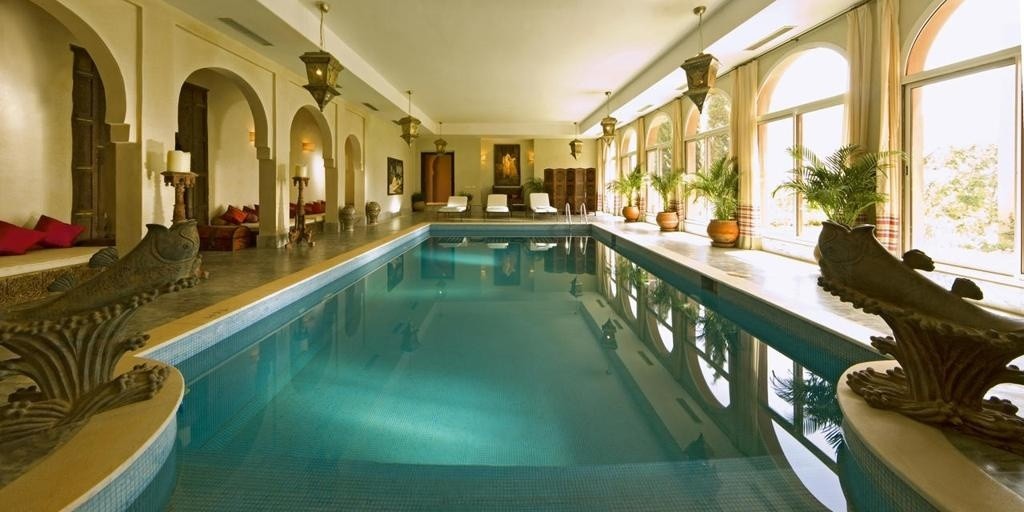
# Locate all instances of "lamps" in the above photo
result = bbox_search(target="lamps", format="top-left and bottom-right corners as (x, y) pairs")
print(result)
(161, 150), (199, 224)
(679, 4), (724, 115)
(434, 121), (448, 156)
(299, 2), (345, 114)
(599, 91), (619, 148)
(285, 164), (316, 249)
(568, 121), (585, 160)
(391, 89), (423, 149)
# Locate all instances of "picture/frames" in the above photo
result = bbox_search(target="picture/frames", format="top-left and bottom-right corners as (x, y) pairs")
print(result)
(493, 144), (520, 186)
(387, 156), (403, 196)
(387, 254), (403, 291)
(493, 243), (521, 287)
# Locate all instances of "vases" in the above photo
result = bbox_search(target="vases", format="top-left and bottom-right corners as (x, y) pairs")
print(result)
(341, 201), (381, 232)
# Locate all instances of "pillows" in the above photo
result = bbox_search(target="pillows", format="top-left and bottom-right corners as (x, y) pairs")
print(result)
(34, 214), (88, 248)
(0, 219), (46, 255)
(212, 200), (325, 225)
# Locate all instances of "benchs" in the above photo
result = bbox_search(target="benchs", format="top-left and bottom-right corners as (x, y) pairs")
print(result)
(241, 212), (326, 241)
(0, 246), (114, 308)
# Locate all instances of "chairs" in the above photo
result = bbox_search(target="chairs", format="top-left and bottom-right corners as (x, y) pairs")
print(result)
(436, 196), (471, 222)
(485, 193), (512, 218)
(529, 193), (558, 222)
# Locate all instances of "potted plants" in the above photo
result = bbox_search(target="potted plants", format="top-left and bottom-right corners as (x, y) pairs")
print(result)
(412, 192), (426, 211)
(772, 142), (910, 261)
(606, 152), (740, 247)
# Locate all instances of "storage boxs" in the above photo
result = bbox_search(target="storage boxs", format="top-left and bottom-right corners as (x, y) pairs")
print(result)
(198, 226), (252, 251)
